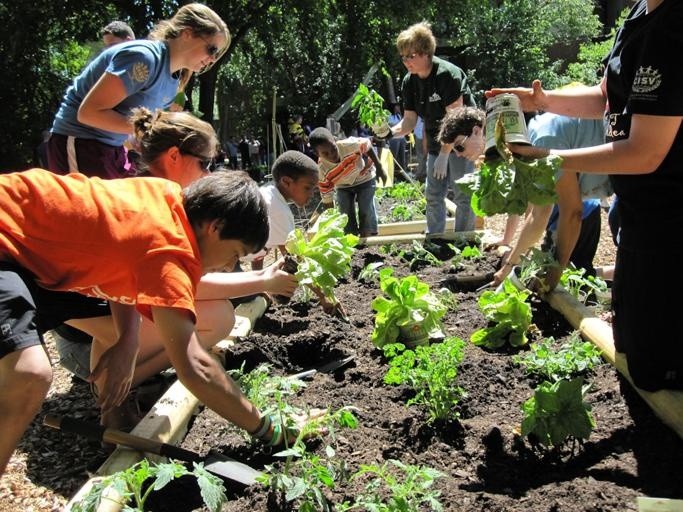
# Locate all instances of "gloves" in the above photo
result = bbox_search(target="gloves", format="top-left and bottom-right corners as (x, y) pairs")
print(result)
(433, 153), (449, 180)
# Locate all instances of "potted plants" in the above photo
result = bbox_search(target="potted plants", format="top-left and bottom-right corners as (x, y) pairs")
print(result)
(453, 92), (563, 220)
(495, 243), (560, 302)
(350, 82), (393, 140)
(273, 208), (360, 304)
(369, 267), (447, 350)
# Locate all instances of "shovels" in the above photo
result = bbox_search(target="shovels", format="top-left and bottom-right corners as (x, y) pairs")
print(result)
(286, 353), (357, 380)
(42, 413), (262, 486)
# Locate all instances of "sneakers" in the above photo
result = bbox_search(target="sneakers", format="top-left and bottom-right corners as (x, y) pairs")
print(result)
(97, 387), (143, 433)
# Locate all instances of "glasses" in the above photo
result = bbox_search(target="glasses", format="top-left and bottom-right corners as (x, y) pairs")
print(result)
(400, 51), (417, 61)
(182, 148), (214, 172)
(454, 136), (470, 152)
(198, 35), (217, 55)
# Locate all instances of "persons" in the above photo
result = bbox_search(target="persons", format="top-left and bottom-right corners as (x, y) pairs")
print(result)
(490, 191), (617, 289)
(378, 18), (478, 232)
(437, 106), (600, 279)
(483, 0), (682, 391)
(44, 4), (425, 388)
(1, 170), (333, 473)
